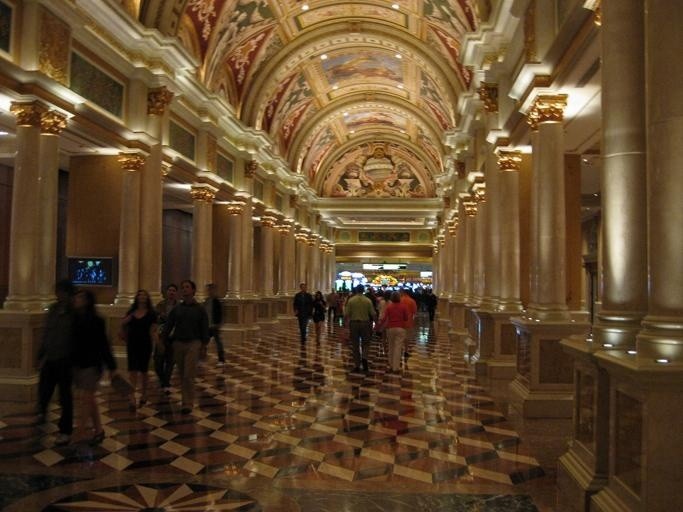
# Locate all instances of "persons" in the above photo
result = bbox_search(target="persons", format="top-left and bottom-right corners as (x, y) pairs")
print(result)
(121, 280), (225, 414)
(51, 292), (119, 445)
(31, 279), (75, 454)
(293, 279), (437, 374)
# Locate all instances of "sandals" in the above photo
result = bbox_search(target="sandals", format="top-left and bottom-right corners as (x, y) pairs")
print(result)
(88, 429), (105, 447)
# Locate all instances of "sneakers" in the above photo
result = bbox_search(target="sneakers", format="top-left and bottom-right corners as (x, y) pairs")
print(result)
(54, 433), (71, 447)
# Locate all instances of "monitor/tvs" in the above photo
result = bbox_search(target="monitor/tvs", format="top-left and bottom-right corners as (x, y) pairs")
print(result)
(66, 255), (115, 290)
